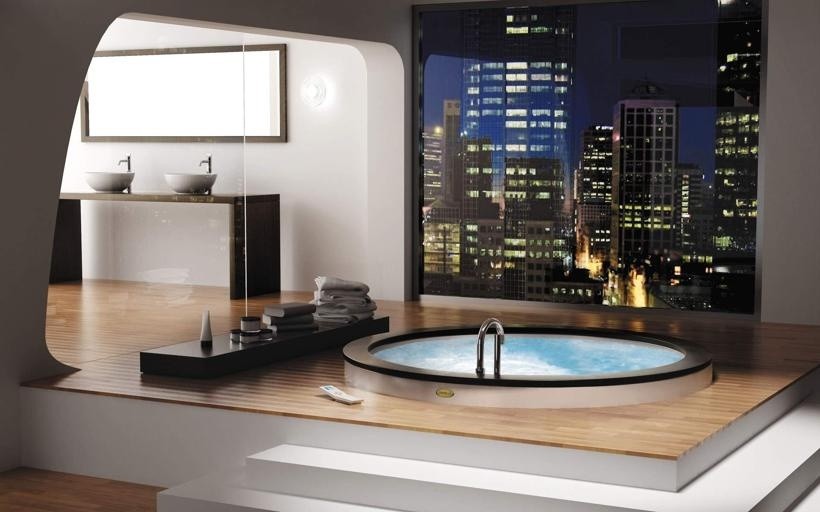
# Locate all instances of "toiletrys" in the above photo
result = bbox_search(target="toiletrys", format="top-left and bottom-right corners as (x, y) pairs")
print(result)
(200, 309), (212, 345)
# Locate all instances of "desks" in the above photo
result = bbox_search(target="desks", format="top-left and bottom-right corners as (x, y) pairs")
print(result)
(48, 191), (279, 300)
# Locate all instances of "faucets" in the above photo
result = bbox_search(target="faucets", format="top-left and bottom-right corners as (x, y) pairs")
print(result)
(476, 317), (504, 376)
(198, 155), (213, 196)
(118, 152), (131, 193)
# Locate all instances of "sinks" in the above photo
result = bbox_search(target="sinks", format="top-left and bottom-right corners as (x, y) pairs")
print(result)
(83, 171), (135, 194)
(162, 173), (217, 196)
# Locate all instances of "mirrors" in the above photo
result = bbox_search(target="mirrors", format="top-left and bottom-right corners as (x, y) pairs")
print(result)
(81, 43), (288, 143)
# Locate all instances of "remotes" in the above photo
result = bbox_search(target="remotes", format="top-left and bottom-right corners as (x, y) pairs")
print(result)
(320, 383), (362, 403)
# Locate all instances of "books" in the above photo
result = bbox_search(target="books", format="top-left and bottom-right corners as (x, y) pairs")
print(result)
(262, 302), (319, 331)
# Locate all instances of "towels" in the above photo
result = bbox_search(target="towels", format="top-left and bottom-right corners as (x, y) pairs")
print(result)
(309, 275), (377, 324)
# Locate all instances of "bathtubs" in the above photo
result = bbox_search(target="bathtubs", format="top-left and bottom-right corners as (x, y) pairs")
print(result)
(338, 320), (715, 410)
